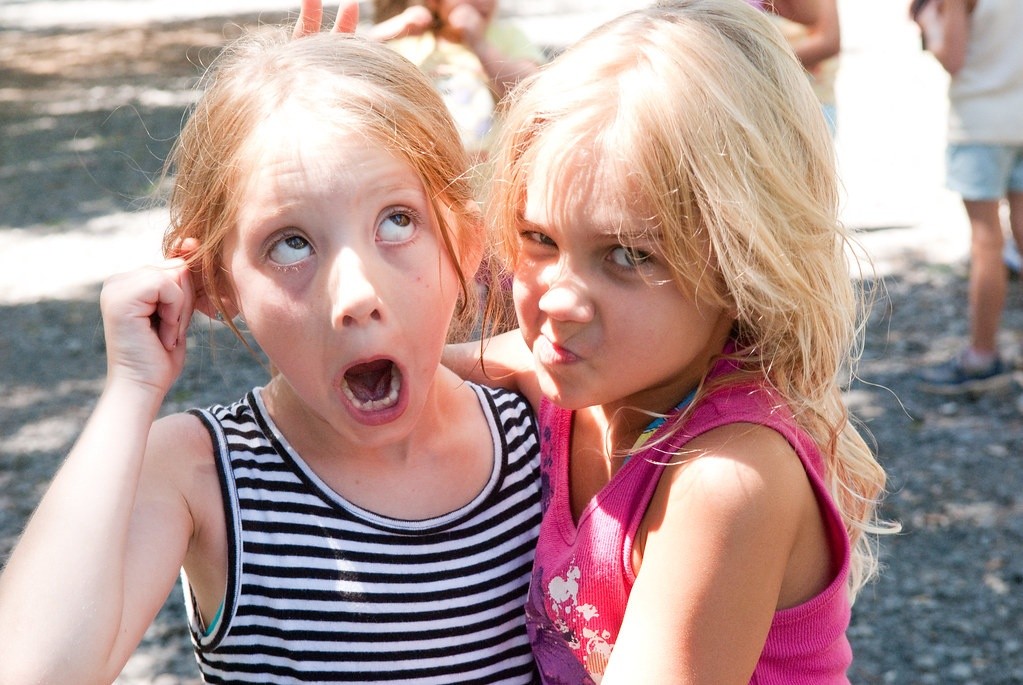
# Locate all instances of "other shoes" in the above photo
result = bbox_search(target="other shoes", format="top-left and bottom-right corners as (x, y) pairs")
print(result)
(917, 351), (1009, 393)
(1002, 236), (1023, 274)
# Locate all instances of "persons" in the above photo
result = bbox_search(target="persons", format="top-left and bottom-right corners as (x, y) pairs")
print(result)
(913, 0), (1023, 395)
(292, 0), (888, 685)
(0, 32), (544, 685)
(373, 0), (546, 149)
(748, 0), (841, 134)
